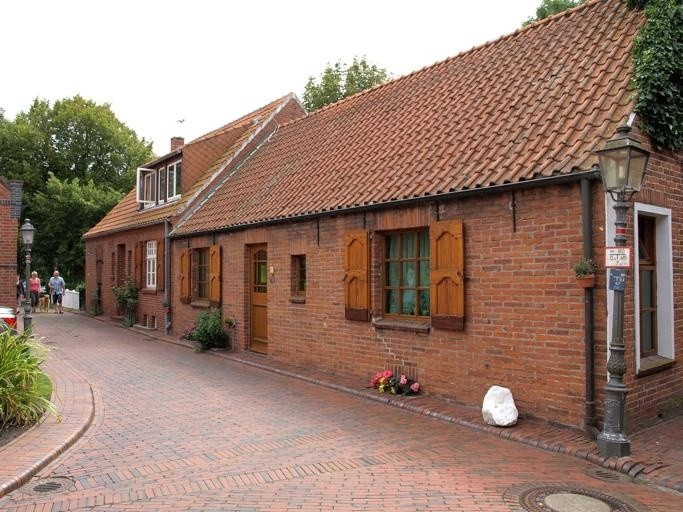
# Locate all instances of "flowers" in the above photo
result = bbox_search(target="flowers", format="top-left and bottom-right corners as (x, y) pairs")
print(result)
(370, 370), (419, 396)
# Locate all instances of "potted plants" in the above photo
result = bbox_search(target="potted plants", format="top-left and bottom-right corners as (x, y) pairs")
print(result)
(573, 257), (597, 287)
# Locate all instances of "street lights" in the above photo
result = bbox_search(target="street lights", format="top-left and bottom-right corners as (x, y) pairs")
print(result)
(19, 218), (37, 339)
(592, 118), (651, 458)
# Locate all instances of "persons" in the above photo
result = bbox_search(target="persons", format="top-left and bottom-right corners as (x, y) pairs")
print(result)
(16, 271), (66, 314)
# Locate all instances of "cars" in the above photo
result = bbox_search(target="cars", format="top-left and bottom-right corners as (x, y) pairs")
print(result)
(19, 278), (47, 298)
